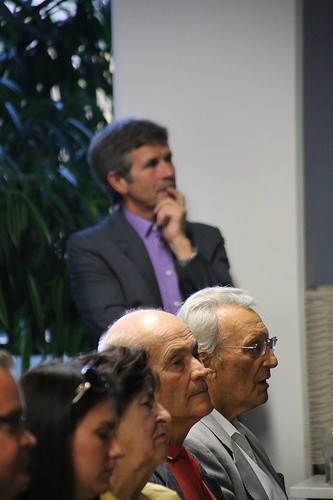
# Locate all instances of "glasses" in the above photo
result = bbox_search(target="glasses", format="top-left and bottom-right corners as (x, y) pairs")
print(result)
(214, 336), (278, 357)
(71, 357), (108, 405)
(0, 410), (25, 435)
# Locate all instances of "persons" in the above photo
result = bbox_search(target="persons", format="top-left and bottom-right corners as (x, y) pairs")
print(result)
(67, 118), (235, 352)
(175, 286), (289, 500)
(0, 345), (182, 500)
(96, 309), (225, 500)
